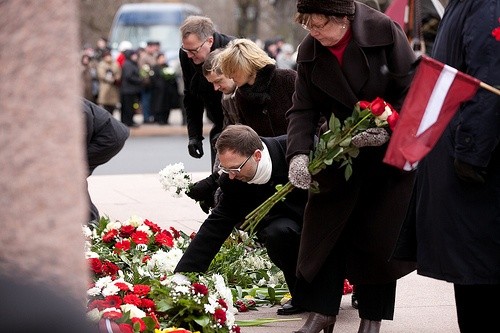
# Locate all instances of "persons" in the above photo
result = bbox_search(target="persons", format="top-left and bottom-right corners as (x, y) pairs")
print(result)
(202, 47), (245, 145)
(213, 38), (298, 138)
(80, 34), (176, 126)
(284, 0), (416, 333)
(83, 94), (131, 227)
(173, 123), (359, 315)
(388, 1), (500, 333)
(253, 0), (450, 73)
(178, 15), (238, 174)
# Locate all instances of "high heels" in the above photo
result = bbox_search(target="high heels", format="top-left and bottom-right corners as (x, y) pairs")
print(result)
(292, 311), (336, 333)
(357, 318), (381, 333)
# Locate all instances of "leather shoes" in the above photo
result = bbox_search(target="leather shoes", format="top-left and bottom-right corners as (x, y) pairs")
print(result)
(351, 292), (358, 308)
(277, 298), (304, 315)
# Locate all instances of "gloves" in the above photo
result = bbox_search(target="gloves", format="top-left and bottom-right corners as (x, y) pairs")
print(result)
(188, 138), (204, 158)
(351, 127), (388, 148)
(288, 154), (311, 190)
(186, 171), (221, 199)
(212, 133), (221, 152)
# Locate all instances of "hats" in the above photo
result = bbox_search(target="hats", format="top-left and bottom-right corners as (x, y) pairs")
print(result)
(297, 0), (356, 16)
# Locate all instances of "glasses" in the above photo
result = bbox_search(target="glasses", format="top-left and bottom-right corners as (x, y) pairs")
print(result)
(218, 154), (253, 174)
(180, 40), (207, 54)
(301, 17), (331, 32)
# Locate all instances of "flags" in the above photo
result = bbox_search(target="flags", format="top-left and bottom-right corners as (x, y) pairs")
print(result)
(383, 53), (479, 170)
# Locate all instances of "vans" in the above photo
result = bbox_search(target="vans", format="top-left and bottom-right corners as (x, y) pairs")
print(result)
(106, 3), (202, 96)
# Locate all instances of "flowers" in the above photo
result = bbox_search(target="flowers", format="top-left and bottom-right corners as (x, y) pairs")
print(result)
(239, 98), (401, 237)
(84, 213), (353, 333)
(160, 67), (178, 80)
(158, 162), (205, 204)
